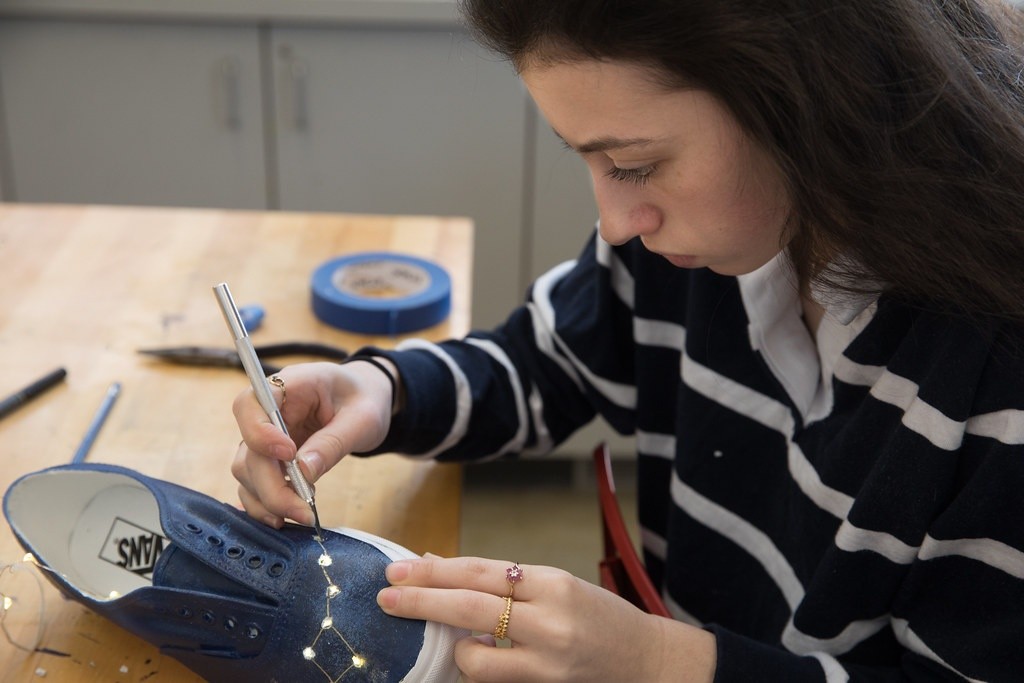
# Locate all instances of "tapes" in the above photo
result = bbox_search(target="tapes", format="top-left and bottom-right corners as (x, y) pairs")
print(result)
(309, 250), (454, 337)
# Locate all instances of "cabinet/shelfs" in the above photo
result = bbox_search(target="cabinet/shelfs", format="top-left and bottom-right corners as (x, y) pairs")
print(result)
(1, 1), (637, 462)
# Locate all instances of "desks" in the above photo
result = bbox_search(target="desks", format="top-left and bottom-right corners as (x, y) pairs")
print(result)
(0, 203), (473, 683)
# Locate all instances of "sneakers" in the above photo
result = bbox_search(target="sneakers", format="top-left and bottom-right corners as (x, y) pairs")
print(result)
(2, 461), (473, 683)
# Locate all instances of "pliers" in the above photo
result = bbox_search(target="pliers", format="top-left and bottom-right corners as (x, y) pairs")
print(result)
(134, 341), (351, 377)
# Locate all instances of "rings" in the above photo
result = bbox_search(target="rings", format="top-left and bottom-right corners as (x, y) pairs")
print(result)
(268, 374), (287, 405)
(506, 562), (522, 597)
(494, 597), (512, 639)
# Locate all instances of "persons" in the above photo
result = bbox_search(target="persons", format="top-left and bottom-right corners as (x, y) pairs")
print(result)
(231, 0), (1024, 683)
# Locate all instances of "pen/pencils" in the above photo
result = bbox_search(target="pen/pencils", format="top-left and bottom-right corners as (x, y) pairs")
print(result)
(72, 379), (122, 465)
(0, 367), (67, 421)
(212, 281), (324, 539)
